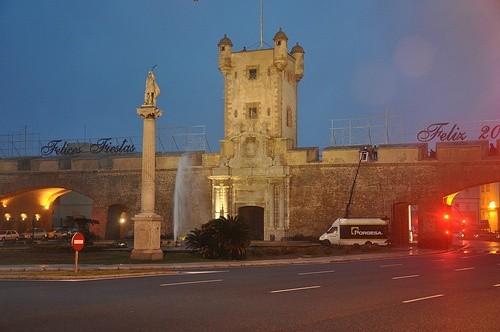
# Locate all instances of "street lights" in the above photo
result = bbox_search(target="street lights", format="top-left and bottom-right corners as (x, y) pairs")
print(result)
(442, 203), (467, 249)
(488, 200), (496, 242)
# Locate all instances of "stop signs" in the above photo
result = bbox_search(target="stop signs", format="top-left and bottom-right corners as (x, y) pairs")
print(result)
(72, 232), (85, 252)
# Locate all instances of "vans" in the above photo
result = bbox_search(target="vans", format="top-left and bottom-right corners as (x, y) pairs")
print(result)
(317, 217), (390, 249)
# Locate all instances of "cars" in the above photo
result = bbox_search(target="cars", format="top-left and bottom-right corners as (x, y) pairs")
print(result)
(0, 229), (19, 241)
(20, 227), (73, 241)
